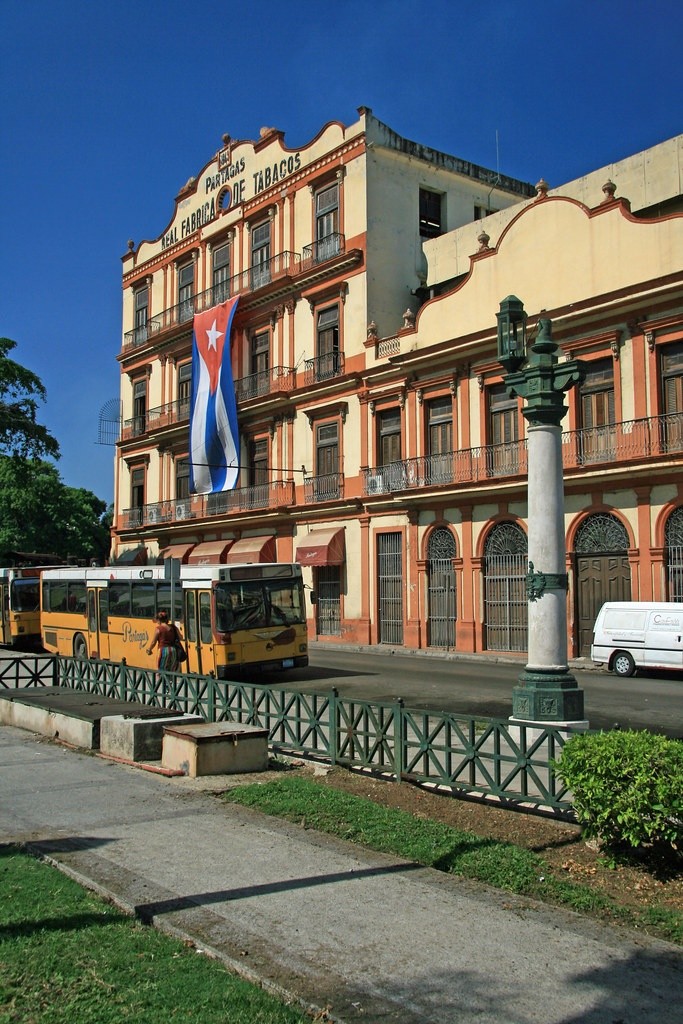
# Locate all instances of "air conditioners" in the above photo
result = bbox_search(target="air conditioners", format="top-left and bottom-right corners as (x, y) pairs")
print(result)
(149, 507), (162, 523)
(369, 475), (389, 495)
(174, 504), (188, 520)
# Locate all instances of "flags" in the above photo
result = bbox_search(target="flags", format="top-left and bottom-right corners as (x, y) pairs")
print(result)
(188, 292), (242, 493)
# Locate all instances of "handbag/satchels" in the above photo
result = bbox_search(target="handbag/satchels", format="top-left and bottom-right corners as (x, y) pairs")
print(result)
(171, 624), (186, 662)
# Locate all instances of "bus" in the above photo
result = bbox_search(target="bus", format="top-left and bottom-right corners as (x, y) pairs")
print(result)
(40, 558), (310, 681)
(0, 565), (79, 647)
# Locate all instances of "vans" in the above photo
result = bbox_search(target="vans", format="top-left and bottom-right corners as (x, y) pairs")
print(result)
(590, 600), (683, 677)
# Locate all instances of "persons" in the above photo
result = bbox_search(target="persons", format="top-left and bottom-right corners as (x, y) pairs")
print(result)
(145, 611), (184, 700)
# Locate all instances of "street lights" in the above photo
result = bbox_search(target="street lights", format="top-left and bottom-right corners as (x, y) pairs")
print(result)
(495, 292), (590, 735)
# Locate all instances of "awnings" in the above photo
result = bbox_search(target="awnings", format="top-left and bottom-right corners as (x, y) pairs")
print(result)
(188, 540), (235, 564)
(156, 543), (197, 565)
(227, 536), (276, 564)
(295, 527), (346, 567)
(114, 548), (149, 566)
